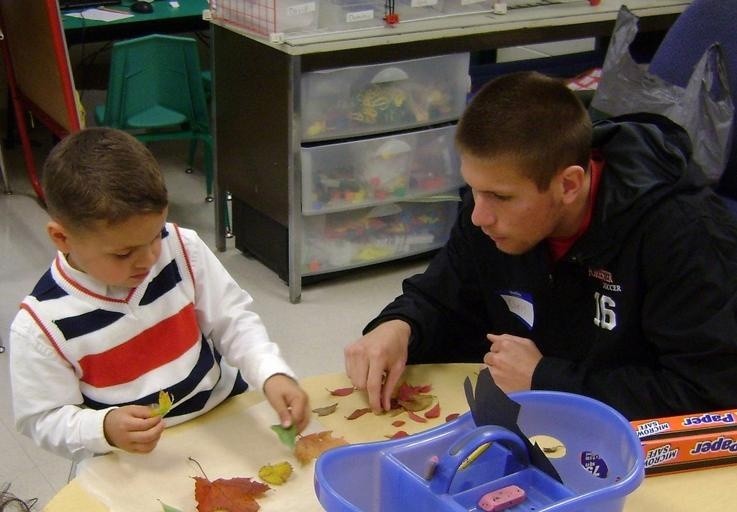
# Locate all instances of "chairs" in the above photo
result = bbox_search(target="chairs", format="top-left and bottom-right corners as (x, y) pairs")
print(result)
(92, 33), (217, 203)
(644, 0), (736, 218)
(185, 63), (218, 199)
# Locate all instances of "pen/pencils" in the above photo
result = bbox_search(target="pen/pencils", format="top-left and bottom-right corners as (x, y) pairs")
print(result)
(456, 441), (495, 472)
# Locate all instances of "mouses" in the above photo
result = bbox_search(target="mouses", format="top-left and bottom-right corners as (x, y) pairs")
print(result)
(130, 1), (152, 13)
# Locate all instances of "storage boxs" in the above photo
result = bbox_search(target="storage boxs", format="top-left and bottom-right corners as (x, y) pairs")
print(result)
(293, 50), (476, 143)
(299, 188), (461, 276)
(301, 124), (470, 217)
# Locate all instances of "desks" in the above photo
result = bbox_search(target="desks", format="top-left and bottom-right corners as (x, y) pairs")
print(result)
(39, 362), (737, 511)
(60, 0), (218, 48)
(202, 0), (737, 302)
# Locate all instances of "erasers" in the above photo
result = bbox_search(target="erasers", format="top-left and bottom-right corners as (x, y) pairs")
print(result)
(479, 485), (527, 512)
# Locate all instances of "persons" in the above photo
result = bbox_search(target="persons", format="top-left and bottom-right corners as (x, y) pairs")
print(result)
(342, 69), (736, 426)
(7, 125), (311, 460)
